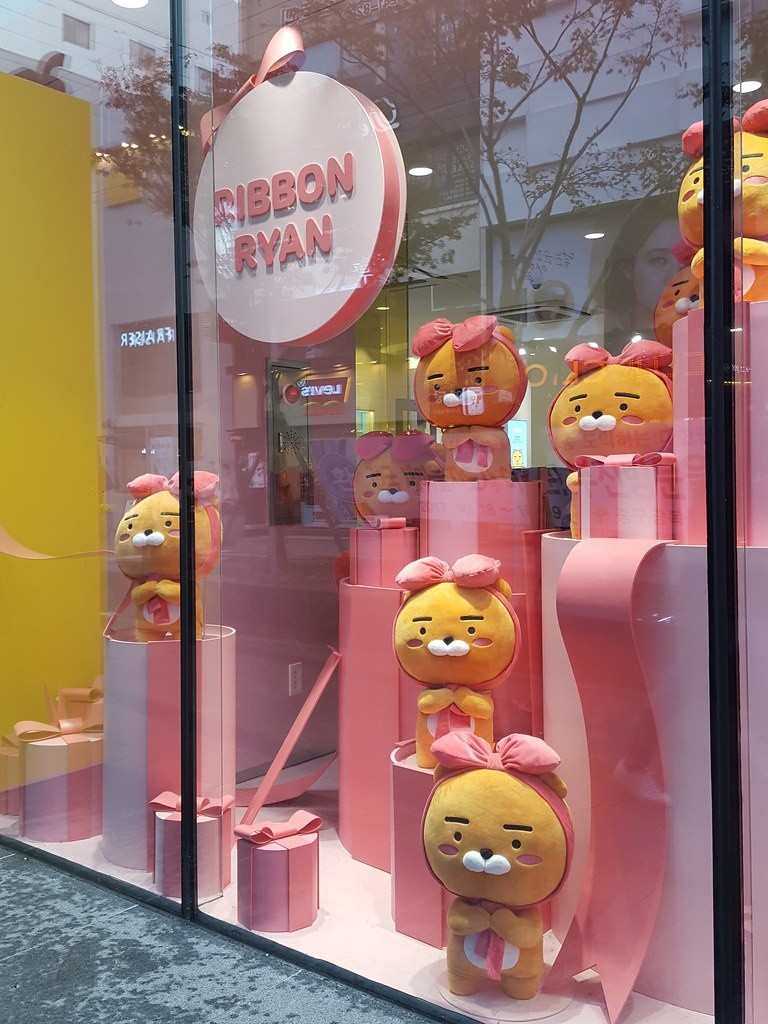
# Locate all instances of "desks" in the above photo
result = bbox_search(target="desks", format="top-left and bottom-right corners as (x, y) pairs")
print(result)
(540, 531), (768, 1024)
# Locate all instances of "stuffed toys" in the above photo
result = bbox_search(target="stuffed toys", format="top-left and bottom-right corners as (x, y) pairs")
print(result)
(113, 471), (223, 642)
(413, 313), (528, 482)
(353, 430), (444, 528)
(419, 729), (574, 999)
(547, 338), (674, 541)
(653, 98), (768, 346)
(392, 554), (521, 768)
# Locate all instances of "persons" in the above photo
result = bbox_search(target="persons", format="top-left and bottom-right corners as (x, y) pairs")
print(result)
(568, 191), (696, 360)
